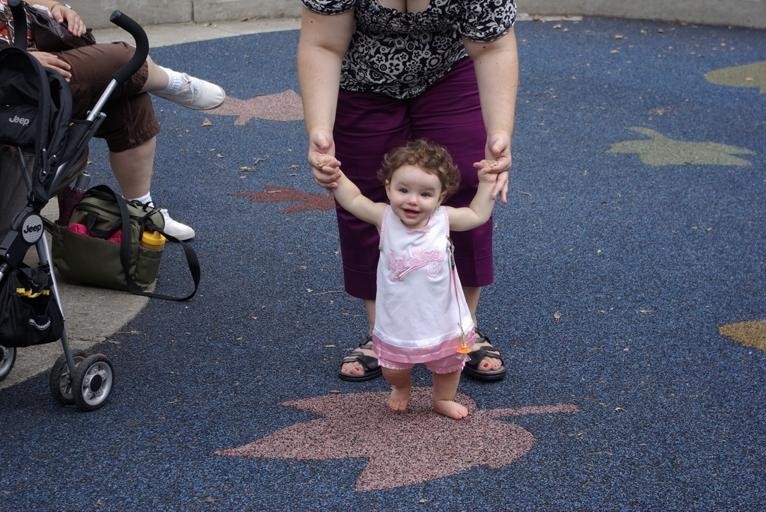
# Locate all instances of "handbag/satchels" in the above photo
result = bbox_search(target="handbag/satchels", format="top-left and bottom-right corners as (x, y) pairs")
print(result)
(50, 184), (202, 305)
(23, 0), (96, 53)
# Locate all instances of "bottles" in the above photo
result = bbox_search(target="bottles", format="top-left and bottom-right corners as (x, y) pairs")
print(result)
(134, 231), (166, 287)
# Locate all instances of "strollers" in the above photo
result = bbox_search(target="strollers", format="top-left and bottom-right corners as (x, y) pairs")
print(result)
(0, 1), (149, 412)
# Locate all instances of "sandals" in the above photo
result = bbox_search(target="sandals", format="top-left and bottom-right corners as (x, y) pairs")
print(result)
(336, 333), (384, 383)
(462, 327), (507, 383)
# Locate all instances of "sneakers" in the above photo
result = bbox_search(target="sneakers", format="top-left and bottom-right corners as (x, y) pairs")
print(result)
(157, 207), (197, 242)
(149, 65), (228, 112)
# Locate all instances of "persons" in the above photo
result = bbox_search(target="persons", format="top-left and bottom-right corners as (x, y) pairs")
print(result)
(316, 138), (498, 420)
(296, 0), (521, 385)
(0, 0), (227, 245)
